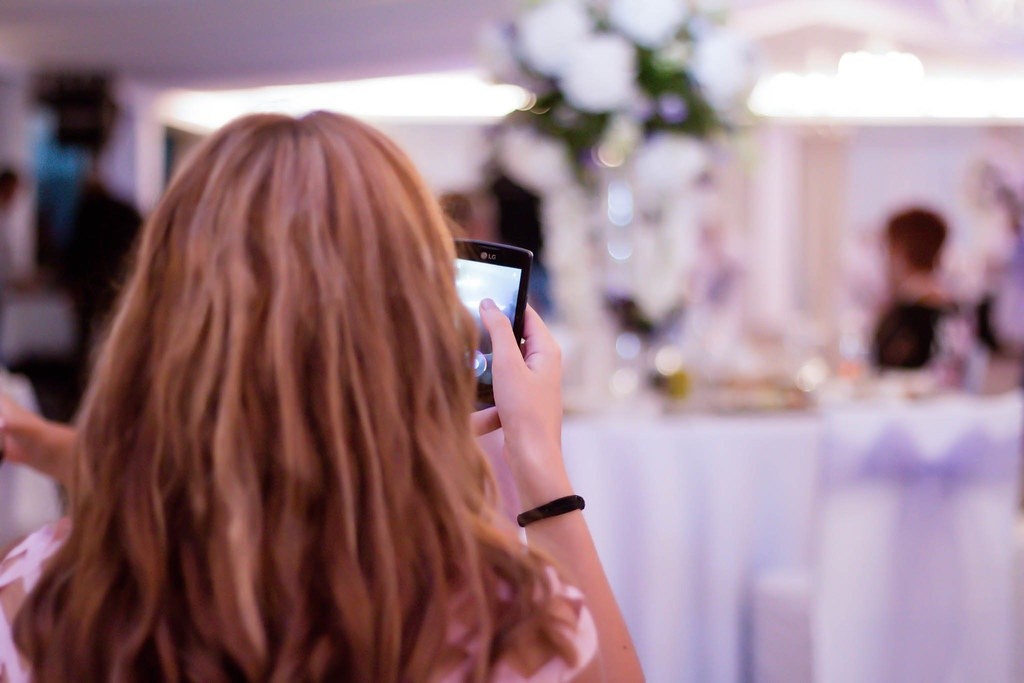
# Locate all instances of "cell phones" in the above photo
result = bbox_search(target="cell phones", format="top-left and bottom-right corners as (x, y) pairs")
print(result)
(450, 239), (534, 403)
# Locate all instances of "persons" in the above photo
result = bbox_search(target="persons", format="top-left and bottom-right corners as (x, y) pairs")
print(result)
(477, 177), (548, 308)
(438, 189), (472, 227)
(4, 125), (147, 366)
(0, 161), (20, 302)
(869, 208), (996, 370)
(0, 111), (643, 683)
